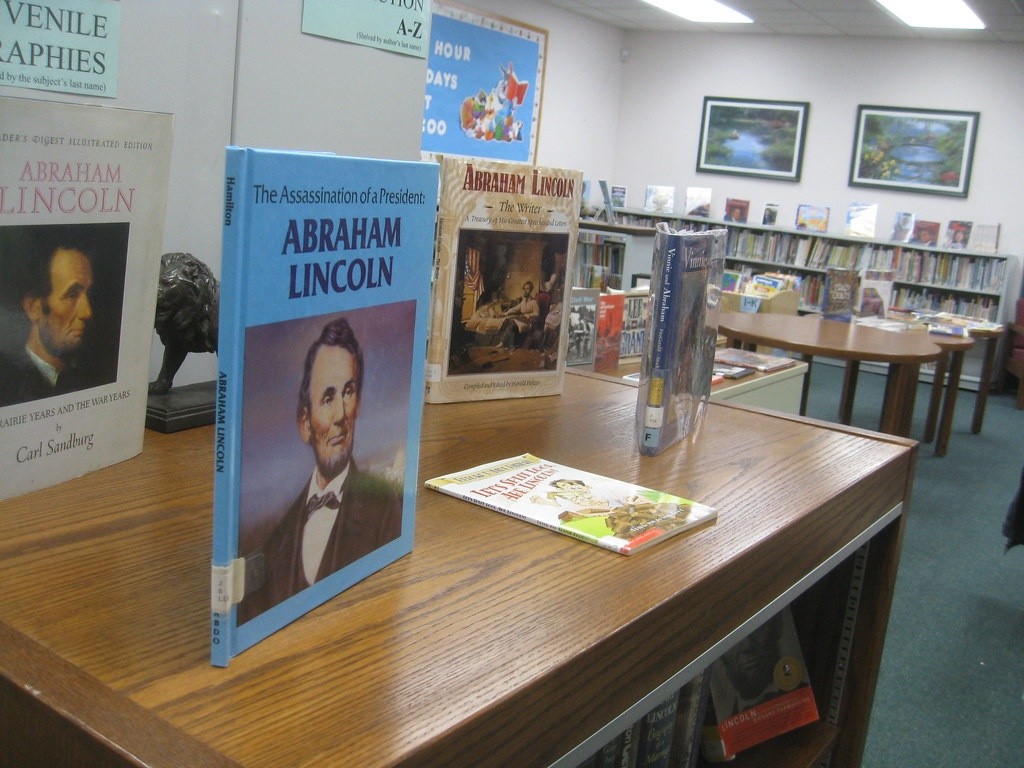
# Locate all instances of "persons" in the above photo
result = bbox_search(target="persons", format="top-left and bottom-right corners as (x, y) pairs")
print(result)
(762, 209), (774, 225)
(724, 206), (746, 223)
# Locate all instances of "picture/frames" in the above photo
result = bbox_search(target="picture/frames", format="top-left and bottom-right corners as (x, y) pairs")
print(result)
(696, 95), (811, 183)
(847, 103), (980, 197)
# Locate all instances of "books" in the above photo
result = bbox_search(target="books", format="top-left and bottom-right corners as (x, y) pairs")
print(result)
(425, 153), (584, 403)
(577, 602), (820, 768)
(623, 347), (796, 384)
(611, 185), (829, 232)
(636, 222), (727, 455)
(0, 95), (174, 499)
(720, 271), (745, 295)
(209, 144), (440, 671)
(844, 201), (877, 237)
(920, 360), (937, 370)
(943, 221), (1001, 253)
(600, 211), (676, 230)
(889, 210), (941, 247)
(565, 286), (650, 371)
(680, 221), (724, 233)
(726, 228), (1007, 338)
(424, 452), (718, 556)
(570, 232), (623, 290)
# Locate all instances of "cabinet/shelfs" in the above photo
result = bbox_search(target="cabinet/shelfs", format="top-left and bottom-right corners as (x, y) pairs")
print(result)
(0, 361), (923, 768)
(567, 206), (1018, 391)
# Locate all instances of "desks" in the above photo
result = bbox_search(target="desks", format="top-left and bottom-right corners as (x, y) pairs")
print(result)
(867, 312), (976, 459)
(923, 305), (1004, 436)
(621, 348), (809, 416)
(719, 313), (940, 439)
